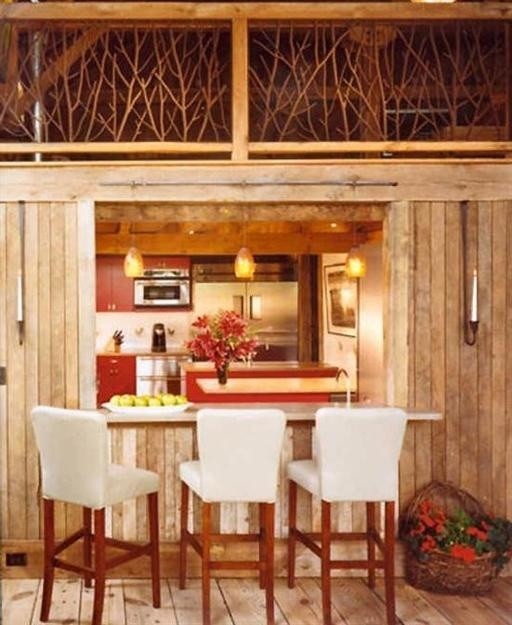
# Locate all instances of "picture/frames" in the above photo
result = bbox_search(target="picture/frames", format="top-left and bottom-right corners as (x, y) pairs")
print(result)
(323, 263), (359, 338)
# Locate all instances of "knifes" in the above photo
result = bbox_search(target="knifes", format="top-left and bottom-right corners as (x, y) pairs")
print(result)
(114, 328), (124, 346)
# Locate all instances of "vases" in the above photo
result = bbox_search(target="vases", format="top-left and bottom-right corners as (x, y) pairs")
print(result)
(214, 361), (228, 384)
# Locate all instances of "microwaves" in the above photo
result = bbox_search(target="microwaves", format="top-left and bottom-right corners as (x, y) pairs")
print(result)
(134, 269), (189, 307)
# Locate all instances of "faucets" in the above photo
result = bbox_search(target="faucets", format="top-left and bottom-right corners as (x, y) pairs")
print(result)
(333, 367), (355, 407)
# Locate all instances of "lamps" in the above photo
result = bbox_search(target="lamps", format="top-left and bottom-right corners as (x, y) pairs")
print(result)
(233, 206), (255, 279)
(346, 203), (366, 278)
(124, 226), (144, 279)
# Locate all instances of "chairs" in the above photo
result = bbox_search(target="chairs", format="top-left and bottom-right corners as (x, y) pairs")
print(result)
(178, 408), (287, 621)
(29, 407), (160, 622)
(288, 405), (409, 622)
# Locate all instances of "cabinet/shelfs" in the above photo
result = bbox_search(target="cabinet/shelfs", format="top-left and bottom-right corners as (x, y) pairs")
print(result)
(96, 257), (133, 311)
(97, 358), (135, 404)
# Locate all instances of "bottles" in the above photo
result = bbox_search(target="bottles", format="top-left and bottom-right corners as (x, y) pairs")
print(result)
(152, 322), (167, 352)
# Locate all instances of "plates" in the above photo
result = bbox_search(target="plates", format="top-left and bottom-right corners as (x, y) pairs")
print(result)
(103, 401), (195, 413)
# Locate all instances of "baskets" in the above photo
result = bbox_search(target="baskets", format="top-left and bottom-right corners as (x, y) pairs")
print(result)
(398, 479), (511, 598)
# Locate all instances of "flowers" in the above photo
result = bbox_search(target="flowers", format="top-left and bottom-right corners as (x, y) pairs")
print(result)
(409, 500), (496, 560)
(187, 310), (260, 367)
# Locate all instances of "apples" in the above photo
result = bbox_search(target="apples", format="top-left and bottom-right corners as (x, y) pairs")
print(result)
(109, 393), (188, 407)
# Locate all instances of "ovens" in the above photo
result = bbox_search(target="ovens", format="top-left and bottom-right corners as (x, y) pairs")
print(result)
(135, 357), (191, 393)
